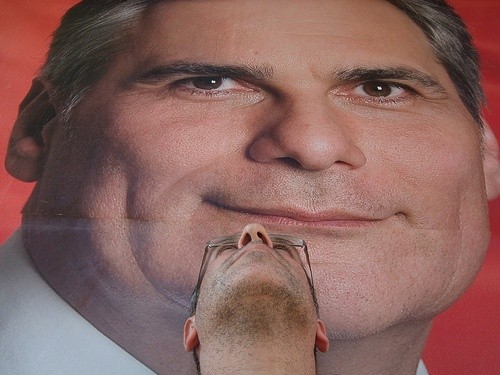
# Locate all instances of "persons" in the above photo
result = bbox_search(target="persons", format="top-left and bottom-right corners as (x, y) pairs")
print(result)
(184, 223), (328, 375)
(3, 1), (499, 375)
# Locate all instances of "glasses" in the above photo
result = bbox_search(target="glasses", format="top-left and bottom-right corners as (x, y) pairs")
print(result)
(193, 233), (316, 308)
